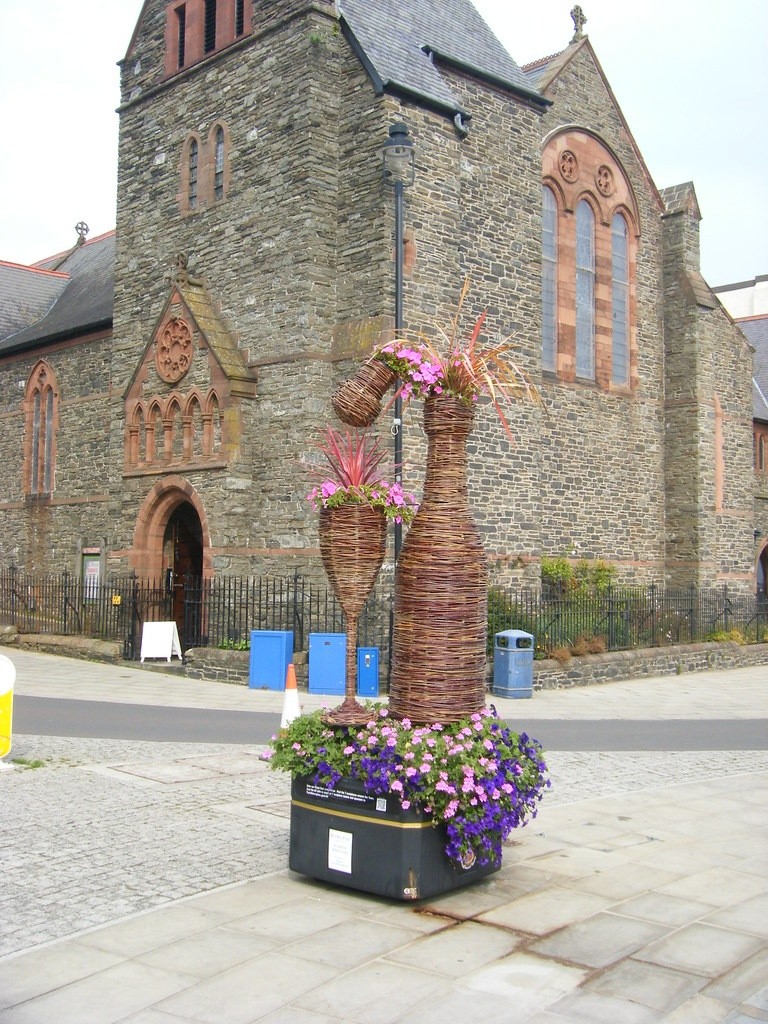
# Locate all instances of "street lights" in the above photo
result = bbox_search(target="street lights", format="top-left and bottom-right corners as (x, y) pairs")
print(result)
(376, 120), (417, 563)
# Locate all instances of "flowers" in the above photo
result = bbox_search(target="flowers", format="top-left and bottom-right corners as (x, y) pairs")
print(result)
(257, 700), (553, 871)
(367, 279), (549, 449)
(296, 426), (422, 526)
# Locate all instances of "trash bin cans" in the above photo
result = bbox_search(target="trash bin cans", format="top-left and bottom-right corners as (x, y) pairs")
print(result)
(0, 655), (16, 758)
(492, 629), (535, 700)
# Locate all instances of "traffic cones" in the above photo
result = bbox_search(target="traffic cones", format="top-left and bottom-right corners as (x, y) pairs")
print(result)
(258, 663), (301, 761)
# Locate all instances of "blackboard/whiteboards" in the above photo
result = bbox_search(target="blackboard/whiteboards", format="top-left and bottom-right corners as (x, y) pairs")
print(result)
(140, 621), (175, 658)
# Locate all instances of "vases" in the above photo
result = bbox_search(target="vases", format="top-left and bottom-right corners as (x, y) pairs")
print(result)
(290, 772), (501, 898)
